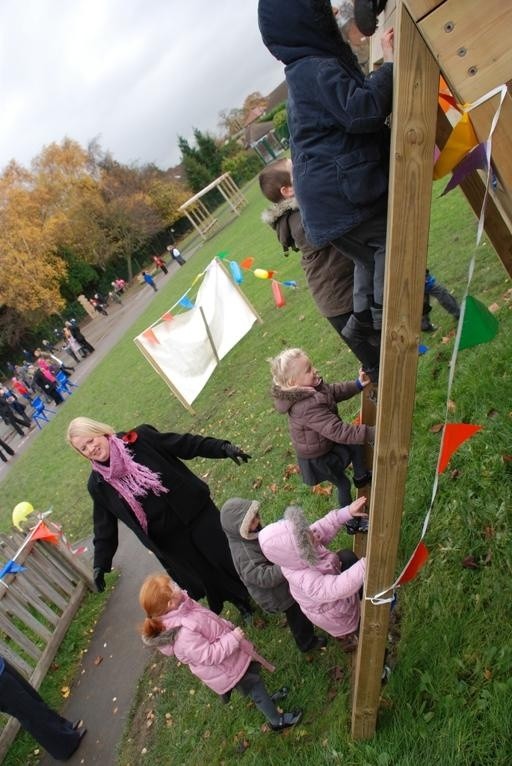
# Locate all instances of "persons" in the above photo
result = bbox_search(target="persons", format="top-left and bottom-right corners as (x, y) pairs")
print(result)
(258, 495), (388, 682)
(139, 573), (304, 730)
(220, 497), (327, 655)
(65, 415), (266, 632)
(256, 0), (393, 339)
(87, 244), (187, 318)
(0, 320), (95, 464)
(265, 346), (372, 534)
(418, 267), (470, 329)
(259, 156), (379, 384)
(0, 652), (88, 761)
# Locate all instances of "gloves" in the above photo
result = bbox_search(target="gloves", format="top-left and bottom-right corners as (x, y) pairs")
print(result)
(220, 442), (252, 466)
(93, 568), (106, 593)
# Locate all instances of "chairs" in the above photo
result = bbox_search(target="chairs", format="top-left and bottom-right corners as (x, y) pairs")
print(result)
(55, 370), (80, 400)
(30, 395), (57, 430)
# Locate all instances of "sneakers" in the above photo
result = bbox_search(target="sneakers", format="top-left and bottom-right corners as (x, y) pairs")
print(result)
(370, 307), (383, 330)
(352, 470), (371, 489)
(346, 518), (369, 534)
(421, 315), (437, 331)
(340, 315), (380, 347)
(301, 636), (326, 652)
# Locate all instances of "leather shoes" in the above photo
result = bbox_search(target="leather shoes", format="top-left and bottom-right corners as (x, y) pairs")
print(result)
(272, 708), (303, 731)
(270, 687), (289, 704)
(72, 720), (80, 729)
(77, 720), (86, 738)
